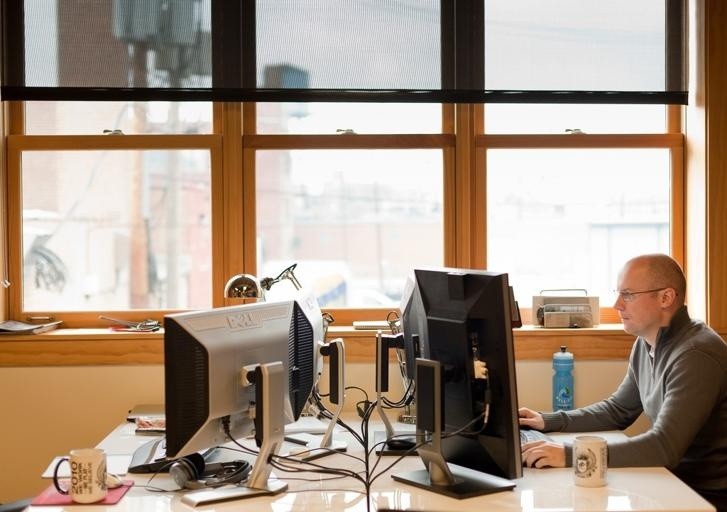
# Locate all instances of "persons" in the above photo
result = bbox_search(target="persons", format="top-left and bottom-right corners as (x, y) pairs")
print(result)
(517, 254), (727, 511)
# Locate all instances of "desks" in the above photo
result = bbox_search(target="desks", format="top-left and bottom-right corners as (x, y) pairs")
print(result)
(22, 412), (714, 506)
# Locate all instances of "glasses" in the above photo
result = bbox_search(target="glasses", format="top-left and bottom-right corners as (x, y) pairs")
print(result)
(611, 288), (661, 303)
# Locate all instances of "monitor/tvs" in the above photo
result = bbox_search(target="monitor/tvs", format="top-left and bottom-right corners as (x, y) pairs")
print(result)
(374, 273), (420, 456)
(163, 298), (297, 508)
(390, 268), (524, 499)
(279, 286), (347, 464)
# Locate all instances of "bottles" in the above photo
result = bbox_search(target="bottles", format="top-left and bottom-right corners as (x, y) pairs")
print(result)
(552, 346), (574, 412)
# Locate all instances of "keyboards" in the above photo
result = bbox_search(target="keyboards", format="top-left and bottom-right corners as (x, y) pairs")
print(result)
(519, 427), (554, 445)
(127, 434), (216, 473)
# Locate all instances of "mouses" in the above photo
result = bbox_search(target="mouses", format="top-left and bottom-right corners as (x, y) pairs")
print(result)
(107, 471), (122, 489)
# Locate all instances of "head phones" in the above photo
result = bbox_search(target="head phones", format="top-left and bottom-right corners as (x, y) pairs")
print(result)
(169, 452), (253, 490)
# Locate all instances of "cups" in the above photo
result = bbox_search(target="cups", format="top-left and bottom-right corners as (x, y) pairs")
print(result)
(572, 436), (608, 487)
(53, 448), (108, 504)
